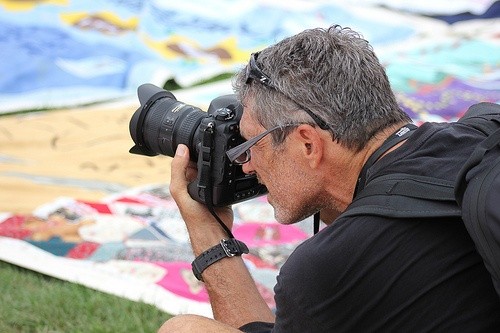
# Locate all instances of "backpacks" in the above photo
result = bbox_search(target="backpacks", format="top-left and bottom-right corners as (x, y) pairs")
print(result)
(328, 104), (500, 293)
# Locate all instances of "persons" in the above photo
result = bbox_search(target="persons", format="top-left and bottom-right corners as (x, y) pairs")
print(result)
(156, 25), (500, 333)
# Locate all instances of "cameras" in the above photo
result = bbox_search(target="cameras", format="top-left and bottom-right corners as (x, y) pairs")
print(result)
(129, 84), (269, 206)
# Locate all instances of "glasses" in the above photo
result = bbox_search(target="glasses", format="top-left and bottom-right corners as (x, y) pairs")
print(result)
(243, 51), (329, 131)
(227, 121), (315, 165)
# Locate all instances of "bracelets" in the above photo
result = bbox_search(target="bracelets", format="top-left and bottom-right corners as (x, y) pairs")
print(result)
(191, 238), (249, 283)
